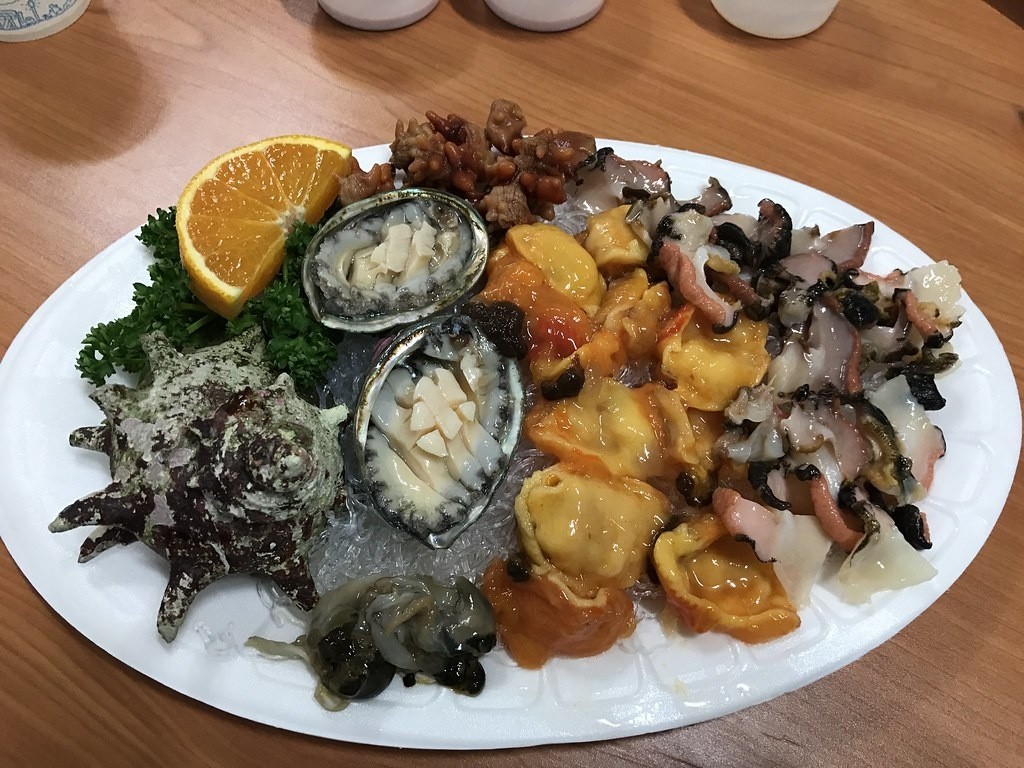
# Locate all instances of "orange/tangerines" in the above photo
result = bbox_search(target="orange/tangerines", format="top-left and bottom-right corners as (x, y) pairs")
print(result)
(175, 134), (352, 322)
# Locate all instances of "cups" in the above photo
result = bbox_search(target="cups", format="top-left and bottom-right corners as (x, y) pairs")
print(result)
(710, 0), (841, 39)
(317, 0), (442, 31)
(484, 0), (609, 32)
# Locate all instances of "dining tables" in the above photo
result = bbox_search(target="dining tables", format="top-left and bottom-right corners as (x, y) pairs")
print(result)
(1, 0), (1024, 768)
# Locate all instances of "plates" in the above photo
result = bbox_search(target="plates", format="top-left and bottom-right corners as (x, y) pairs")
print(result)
(0, 0), (92, 43)
(1, 134), (1023, 750)
